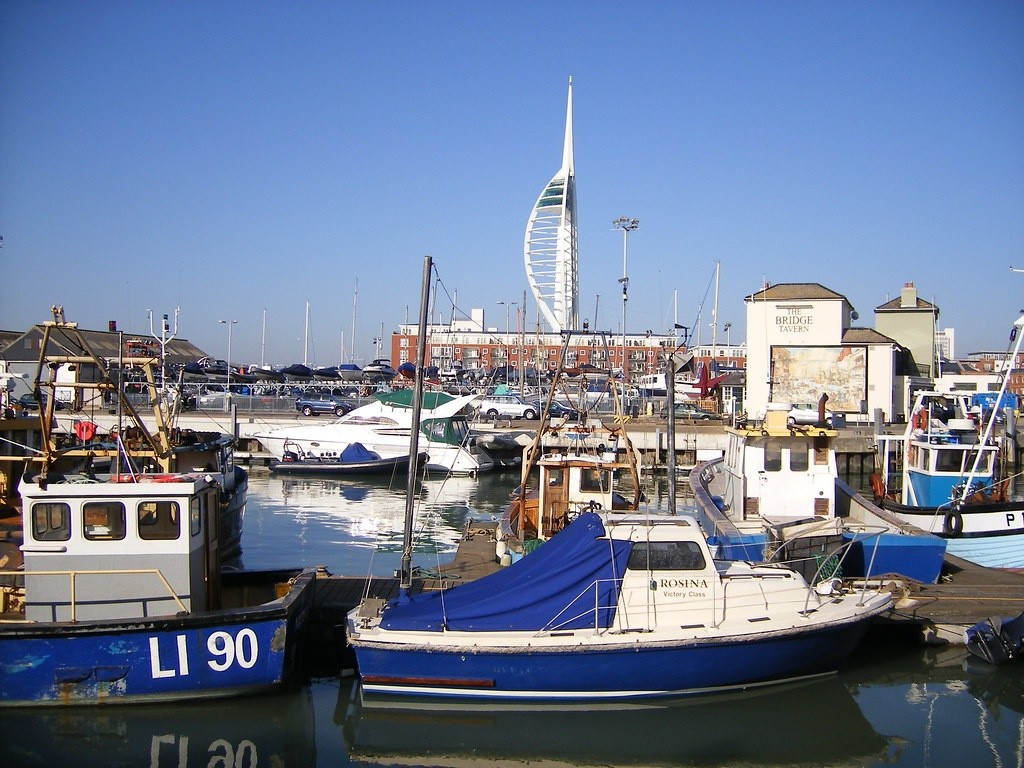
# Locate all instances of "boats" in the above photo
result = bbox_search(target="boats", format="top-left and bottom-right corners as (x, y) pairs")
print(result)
(869, 265), (1023, 569)
(343, 513), (898, 705)
(0, 386), (494, 573)
(495, 328), (641, 567)
(333, 675), (907, 768)
(0, 470), (318, 707)
(0, 673), (317, 768)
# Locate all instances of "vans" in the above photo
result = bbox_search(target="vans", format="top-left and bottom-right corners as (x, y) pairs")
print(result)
(983, 408), (1007, 424)
(787, 403), (839, 428)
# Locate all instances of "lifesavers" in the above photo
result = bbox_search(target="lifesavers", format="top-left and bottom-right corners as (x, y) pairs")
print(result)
(912, 404), (927, 437)
(944, 509), (963, 537)
(135, 475), (194, 483)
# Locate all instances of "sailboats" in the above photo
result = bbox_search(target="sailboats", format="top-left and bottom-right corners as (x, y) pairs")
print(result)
(175, 277), (728, 397)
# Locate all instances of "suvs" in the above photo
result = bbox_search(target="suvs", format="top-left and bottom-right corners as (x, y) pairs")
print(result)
(295, 394), (356, 417)
(479, 396), (540, 420)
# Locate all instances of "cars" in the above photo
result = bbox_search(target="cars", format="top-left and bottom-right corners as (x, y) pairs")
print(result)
(20, 394), (65, 412)
(537, 399), (578, 421)
(660, 403), (720, 421)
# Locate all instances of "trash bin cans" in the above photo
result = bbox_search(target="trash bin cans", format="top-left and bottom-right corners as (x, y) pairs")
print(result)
(832, 413), (846, 429)
(626, 406), (638, 418)
(897, 413), (905, 424)
